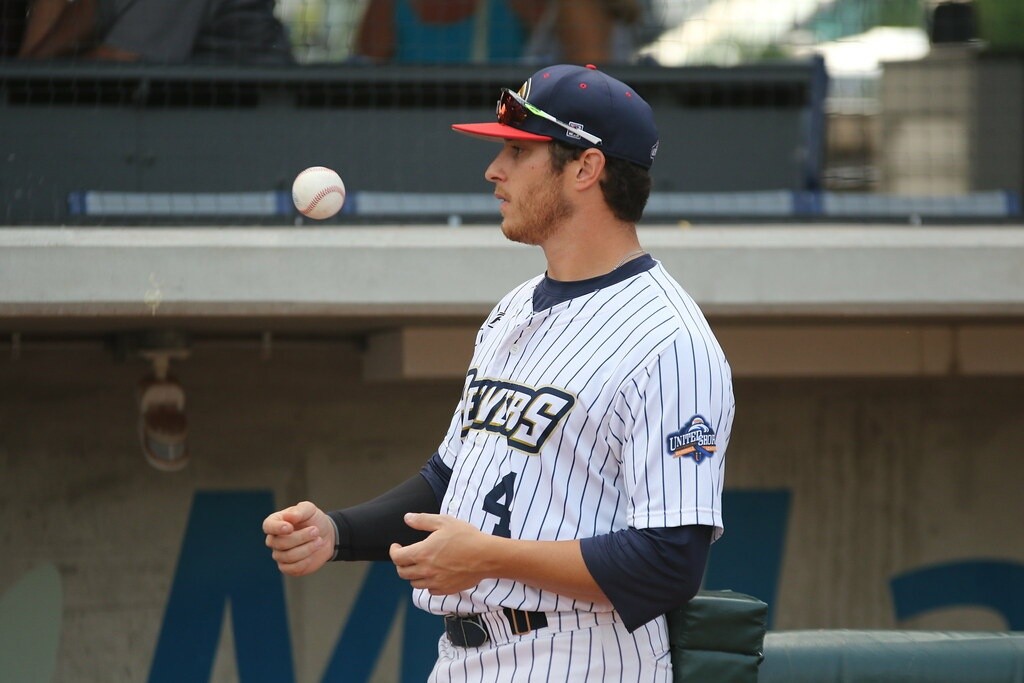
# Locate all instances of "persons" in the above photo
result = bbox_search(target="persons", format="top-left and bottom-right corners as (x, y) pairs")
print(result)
(263, 64), (737, 683)
(1, 0), (665, 65)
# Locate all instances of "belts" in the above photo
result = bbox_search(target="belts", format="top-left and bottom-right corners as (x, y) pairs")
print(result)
(445, 607), (549, 649)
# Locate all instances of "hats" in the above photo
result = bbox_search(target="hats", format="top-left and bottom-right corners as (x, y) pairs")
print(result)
(451, 63), (658, 168)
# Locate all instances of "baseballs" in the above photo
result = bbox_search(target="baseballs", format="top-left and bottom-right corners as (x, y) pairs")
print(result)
(290, 163), (350, 221)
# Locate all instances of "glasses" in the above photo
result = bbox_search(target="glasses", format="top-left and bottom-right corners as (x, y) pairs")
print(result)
(496, 87), (602, 148)
